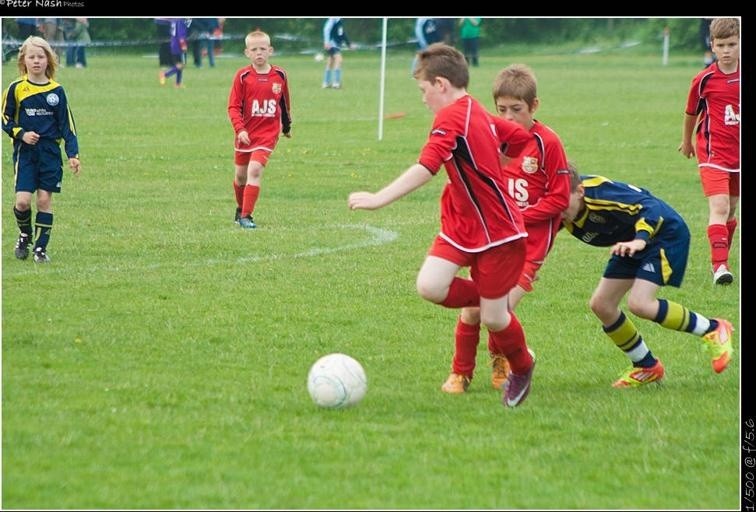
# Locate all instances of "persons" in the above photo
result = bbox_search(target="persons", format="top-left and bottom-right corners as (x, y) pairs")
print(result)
(345, 39), (539, 410)
(699, 18), (718, 68)
(2, 34), (82, 264)
(4, 18), (89, 71)
(676, 18), (740, 285)
(319, 18), (355, 89)
(154, 18), (227, 91)
(227, 29), (296, 229)
(437, 62), (571, 395)
(559, 162), (737, 389)
(458, 18), (484, 68)
(411, 18), (459, 80)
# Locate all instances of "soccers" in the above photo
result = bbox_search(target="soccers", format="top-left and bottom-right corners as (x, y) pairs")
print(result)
(308, 354), (366, 408)
(314, 52), (325, 63)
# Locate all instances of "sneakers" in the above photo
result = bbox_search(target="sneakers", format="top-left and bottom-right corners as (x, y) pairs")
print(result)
(235, 208), (256, 228)
(700, 318), (735, 374)
(713, 264), (734, 285)
(441, 348), (536, 409)
(15, 232), (50, 263)
(611, 359), (665, 388)
